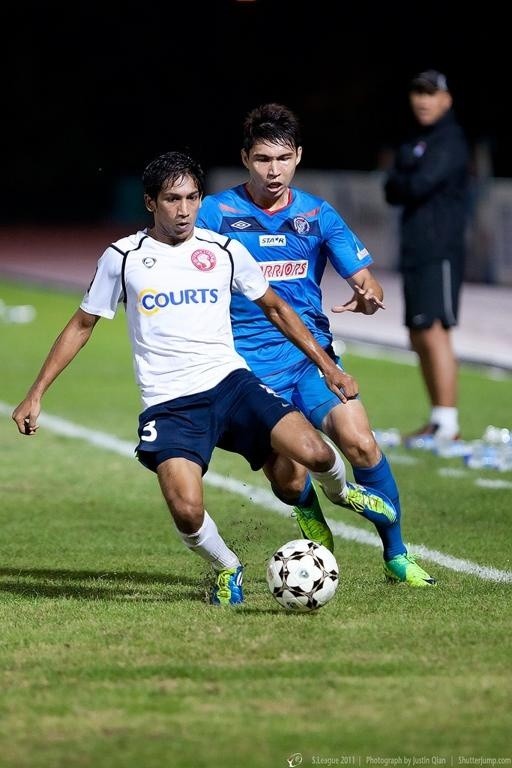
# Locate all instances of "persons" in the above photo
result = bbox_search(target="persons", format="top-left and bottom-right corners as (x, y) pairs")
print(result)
(12, 151), (359, 606)
(195, 103), (436, 587)
(383, 69), (471, 451)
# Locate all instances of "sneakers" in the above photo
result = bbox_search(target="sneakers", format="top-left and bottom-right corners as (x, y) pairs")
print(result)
(403, 422), (460, 447)
(209, 548), (244, 607)
(292, 506), (334, 555)
(318, 480), (396, 525)
(382, 543), (437, 589)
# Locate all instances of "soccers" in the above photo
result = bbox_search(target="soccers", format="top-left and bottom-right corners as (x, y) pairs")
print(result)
(265, 539), (340, 615)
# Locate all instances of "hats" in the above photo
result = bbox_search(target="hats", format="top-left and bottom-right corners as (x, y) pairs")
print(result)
(412, 70), (448, 94)
